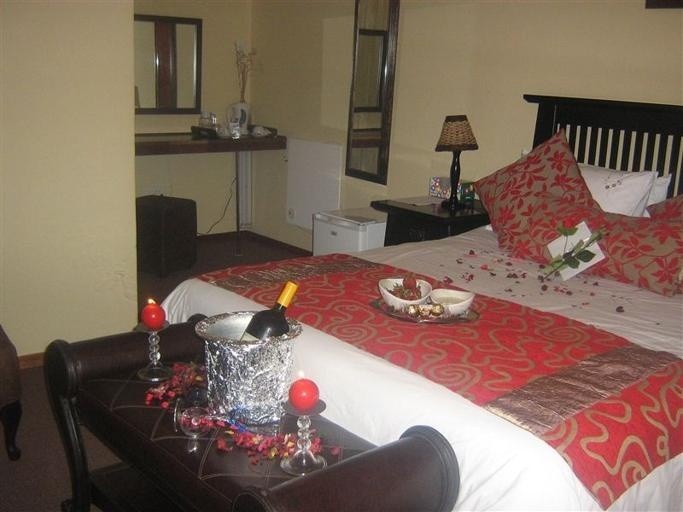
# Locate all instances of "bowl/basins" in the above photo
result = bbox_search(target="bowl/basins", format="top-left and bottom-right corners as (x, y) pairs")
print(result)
(430, 288), (476, 316)
(378, 278), (434, 311)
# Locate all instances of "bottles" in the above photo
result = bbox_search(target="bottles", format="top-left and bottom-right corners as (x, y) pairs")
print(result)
(241, 280), (298, 342)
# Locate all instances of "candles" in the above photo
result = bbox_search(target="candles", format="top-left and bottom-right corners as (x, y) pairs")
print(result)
(139, 297), (166, 330)
(289, 368), (320, 413)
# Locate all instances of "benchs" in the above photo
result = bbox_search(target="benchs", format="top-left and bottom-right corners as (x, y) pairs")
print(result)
(44, 313), (462, 511)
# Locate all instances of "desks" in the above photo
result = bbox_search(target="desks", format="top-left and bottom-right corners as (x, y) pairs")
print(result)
(135, 126), (286, 249)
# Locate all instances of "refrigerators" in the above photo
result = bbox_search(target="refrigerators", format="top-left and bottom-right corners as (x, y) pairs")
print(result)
(313, 205), (387, 262)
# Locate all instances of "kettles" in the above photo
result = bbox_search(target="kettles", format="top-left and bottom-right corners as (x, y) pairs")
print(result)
(227, 102), (250, 134)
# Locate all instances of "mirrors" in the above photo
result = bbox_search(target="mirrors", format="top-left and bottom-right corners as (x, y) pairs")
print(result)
(352, 28), (386, 113)
(134, 14), (203, 115)
(345, 0), (400, 186)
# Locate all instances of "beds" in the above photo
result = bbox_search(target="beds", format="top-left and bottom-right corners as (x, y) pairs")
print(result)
(169, 91), (683, 512)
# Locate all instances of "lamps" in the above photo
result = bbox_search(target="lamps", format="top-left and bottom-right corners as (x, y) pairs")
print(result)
(434, 115), (479, 208)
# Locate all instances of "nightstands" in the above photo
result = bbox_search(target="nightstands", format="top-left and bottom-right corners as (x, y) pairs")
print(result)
(370, 192), (489, 246)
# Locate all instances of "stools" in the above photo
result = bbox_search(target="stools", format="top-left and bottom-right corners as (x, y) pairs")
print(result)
(136, 194), (197, 279)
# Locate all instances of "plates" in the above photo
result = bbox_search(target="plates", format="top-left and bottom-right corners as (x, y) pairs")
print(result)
(373, 297), (480, 324)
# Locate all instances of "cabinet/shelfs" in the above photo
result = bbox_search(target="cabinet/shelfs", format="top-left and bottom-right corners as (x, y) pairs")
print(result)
(312, 207), (387, 256)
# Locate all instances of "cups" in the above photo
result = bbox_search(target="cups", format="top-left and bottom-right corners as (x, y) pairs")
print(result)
(199, 110), (240, 141)
(194, 312), (302, 427)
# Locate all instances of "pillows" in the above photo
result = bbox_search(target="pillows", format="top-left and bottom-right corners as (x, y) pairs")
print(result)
(470, 127), (683, 298)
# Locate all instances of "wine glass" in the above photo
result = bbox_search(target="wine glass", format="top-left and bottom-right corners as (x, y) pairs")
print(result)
(181, 364), (212, 440)
